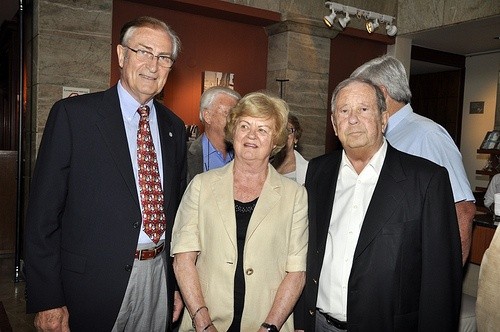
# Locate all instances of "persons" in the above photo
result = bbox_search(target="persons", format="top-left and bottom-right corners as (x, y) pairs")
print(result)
(186, 86), (244, 185)
(269, 115), (311, 185)
(351, 56), (478, 279)
(19, 16), (189, 332)
(168, 92), (309, 332)
(483, 172), (500, 212)
(292, 76), (465, 332)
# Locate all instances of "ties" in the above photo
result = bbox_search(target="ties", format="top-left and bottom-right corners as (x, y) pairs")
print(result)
(136, 104), (166, 244)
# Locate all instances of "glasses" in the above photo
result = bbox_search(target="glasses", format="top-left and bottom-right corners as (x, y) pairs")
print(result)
(288, 128), (297, 135)
(126, 45), (177, 68)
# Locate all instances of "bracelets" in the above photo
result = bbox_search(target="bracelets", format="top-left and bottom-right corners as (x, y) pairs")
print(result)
(191, 306), (208, 328)
(203, 324), (213, 331)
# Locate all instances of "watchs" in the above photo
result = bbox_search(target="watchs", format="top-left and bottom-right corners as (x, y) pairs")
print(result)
(261, 323), (279, 332)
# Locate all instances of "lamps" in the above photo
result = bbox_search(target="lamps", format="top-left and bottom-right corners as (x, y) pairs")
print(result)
(386, 21), (398, 37)
(365, 17), (380, 33)
(324, 9), (337, 27)
(338, 12), (350, 28)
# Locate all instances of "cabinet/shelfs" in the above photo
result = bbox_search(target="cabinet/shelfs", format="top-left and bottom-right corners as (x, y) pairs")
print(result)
(473, 129), (500, 213)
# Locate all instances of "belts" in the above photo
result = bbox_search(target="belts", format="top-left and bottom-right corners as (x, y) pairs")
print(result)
(134, 242), (165, 263)
(315, 307), (348, 330)
